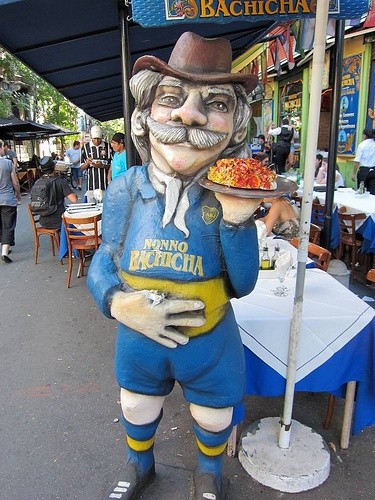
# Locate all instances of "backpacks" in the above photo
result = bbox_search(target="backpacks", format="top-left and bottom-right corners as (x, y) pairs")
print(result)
(30, 178), (60, 215)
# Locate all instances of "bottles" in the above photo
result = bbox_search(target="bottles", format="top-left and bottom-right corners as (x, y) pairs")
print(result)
(261, 247), (270, 270)
(271, 246), (280, 270)
(359, 180), (367, 194)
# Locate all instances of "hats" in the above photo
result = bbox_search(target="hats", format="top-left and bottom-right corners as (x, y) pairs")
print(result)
(91, 126), (102, 139)
(112, 133), (124, 144)
(39, 157), (57, 170)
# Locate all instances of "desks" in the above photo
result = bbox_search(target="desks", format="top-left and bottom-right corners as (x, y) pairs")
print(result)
(276, 174), (338, 192)
(293, 187), (375, 285)
(255, 232), (316, 268)
(58, 202), (112, 265)
(227, 268), (375, 458)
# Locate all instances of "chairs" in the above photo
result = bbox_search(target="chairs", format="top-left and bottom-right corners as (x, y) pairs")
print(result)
(26, 203), (70, 267)
(27, 168), (40, 189)
(17, 172), (31, 193)
(84, 191), (94, 203)
(289, 237), (332, 272)
(312, 203), (326, 231)
(337, 206), (367, 285)
(366, 269), (375, 282)
(61, 213), (105, 289)
(290, 196), (302, 209)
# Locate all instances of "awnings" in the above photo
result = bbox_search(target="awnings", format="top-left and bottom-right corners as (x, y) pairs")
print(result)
(1, 0), (368, 254)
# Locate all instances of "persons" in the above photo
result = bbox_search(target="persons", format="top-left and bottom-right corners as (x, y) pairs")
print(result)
(86, 30), (263, 500)
(0, 120), (374, 265)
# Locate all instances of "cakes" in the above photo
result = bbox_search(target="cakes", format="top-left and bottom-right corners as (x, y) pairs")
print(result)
(208, 157), (278, 190)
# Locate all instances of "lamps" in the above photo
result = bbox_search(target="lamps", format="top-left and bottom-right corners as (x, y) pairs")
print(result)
(93, 189), (104, 205)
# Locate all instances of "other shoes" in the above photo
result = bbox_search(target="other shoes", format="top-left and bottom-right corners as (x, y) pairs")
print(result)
(8, 249), (11, 254)
(78, 186), (82, 191)
(2, 255), (11, 263)
(72, 250), (91, 258)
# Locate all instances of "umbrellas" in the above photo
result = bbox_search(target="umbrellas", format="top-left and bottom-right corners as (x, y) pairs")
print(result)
(0, 115), (87, 160)
